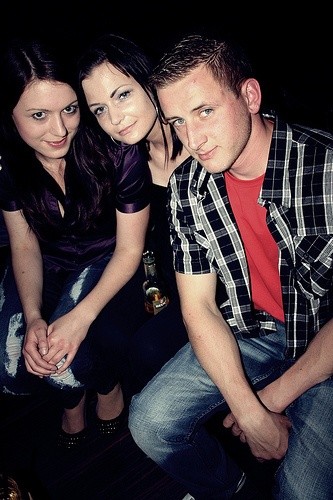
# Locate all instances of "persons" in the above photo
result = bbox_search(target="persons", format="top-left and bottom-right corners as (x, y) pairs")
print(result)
(128, 33), (333, 500)
(1, 32), (228, 500)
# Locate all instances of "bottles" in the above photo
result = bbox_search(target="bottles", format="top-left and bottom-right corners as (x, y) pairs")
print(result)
(142, 251), (170, 317)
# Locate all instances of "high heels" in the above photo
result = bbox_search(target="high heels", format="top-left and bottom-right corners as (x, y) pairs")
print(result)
(93, 386), (126, 443)
(51, 385), (89, 465)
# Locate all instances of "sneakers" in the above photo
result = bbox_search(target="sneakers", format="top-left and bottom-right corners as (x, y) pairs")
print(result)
(182, 471), (248, 500)
